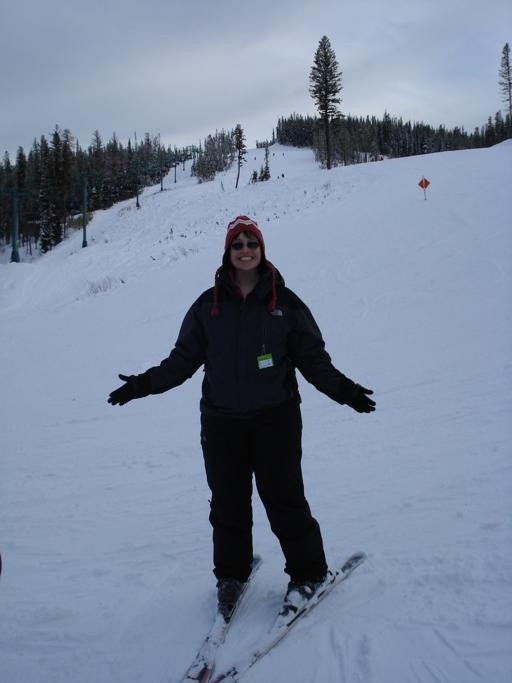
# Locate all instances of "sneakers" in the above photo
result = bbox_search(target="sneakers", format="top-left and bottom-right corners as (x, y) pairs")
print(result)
(215, 576), (242, 615)
(277, 570), (337, 625)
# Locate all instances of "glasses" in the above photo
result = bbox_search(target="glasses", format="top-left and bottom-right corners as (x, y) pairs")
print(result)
(231, 241), (258, 249)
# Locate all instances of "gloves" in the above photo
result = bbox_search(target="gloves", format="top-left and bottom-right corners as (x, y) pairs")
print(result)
(107, 372), (140, 405)
(345, 383), (376, 413)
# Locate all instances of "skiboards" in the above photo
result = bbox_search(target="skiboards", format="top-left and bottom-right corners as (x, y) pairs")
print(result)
(183, 551), (371, 682)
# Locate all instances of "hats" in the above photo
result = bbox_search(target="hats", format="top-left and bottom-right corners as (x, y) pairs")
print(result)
(224, 215), (265, 262)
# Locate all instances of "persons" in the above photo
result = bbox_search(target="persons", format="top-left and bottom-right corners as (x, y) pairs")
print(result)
(107, 216), (376, 604)
